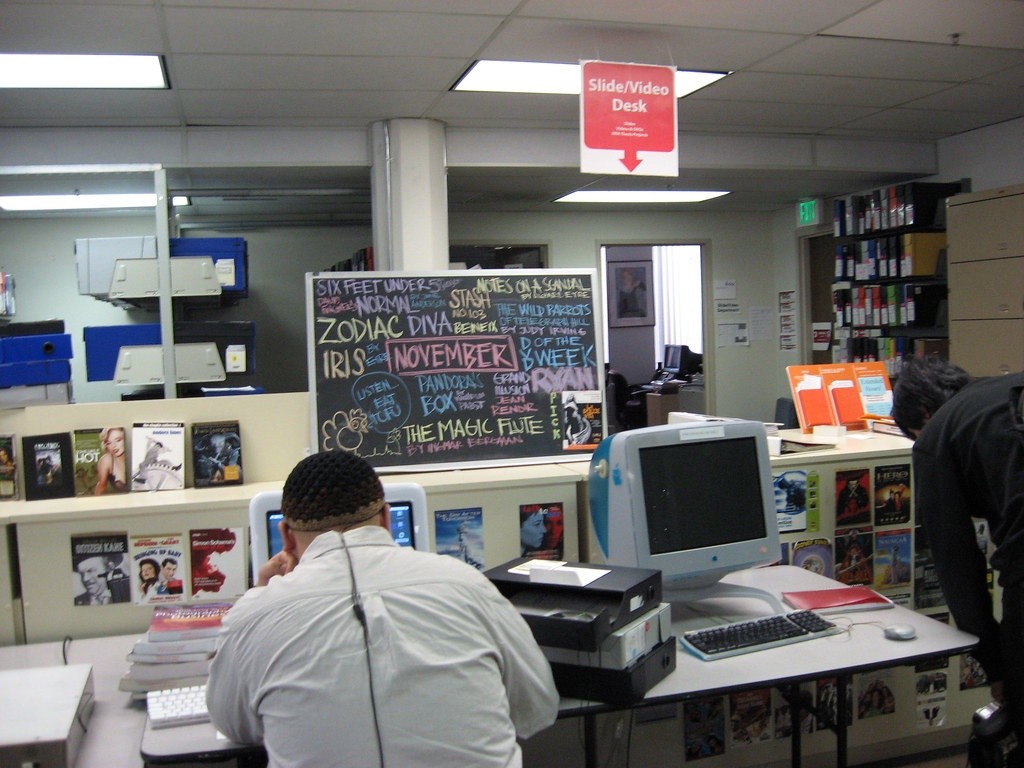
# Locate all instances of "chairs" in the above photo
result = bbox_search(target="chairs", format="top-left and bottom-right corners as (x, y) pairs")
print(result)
(606, 368), (647, 431)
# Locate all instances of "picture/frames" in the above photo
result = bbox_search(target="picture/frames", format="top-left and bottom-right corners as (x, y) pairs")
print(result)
(607, 260), (655, 328)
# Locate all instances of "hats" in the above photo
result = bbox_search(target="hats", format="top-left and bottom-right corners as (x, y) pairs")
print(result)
(281, 451), (386, 531)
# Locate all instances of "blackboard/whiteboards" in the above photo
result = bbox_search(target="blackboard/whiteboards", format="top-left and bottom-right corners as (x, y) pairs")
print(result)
(306, 267), (608, 473)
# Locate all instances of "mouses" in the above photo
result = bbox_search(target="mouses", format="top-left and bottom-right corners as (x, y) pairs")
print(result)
(884, 624), (916, 639)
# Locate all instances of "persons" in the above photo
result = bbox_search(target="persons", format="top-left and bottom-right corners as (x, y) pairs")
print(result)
(891, 355), (1024, 768)
(72, 552), (125, 606)
(915, 672), (946, 720)
(876, 483), (908, 525)
(520, 506), (563, 559)
(684, 684), (852, 763)
(92, 425), (125, 496)
(858, 679), (895, 720)
(205, 450), (560, 768)
(0, 447), (15, 474)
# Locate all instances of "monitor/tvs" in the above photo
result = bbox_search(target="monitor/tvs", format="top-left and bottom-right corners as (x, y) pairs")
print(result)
(588, 420), (785, 615)
(249, 482), (429, 588)
(664, 344), (703, 381)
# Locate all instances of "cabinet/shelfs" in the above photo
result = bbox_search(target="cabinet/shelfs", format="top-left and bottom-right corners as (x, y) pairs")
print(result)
(944, 184), (1023, 379)
(827, 178), (972, 367)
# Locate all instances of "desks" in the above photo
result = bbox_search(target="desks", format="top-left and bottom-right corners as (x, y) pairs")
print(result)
(141, 558), (979, 768)
(0, 632), (146, 768)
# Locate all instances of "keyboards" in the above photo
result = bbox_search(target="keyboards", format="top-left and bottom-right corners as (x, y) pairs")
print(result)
(145, 684), (211, 730)
(679, 609), (842, 662)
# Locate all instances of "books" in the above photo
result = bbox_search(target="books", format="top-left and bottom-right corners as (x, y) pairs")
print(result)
(119, 604), (235, 710)
(0, 272), (16, 316)
(781, 584), (895, 616)
